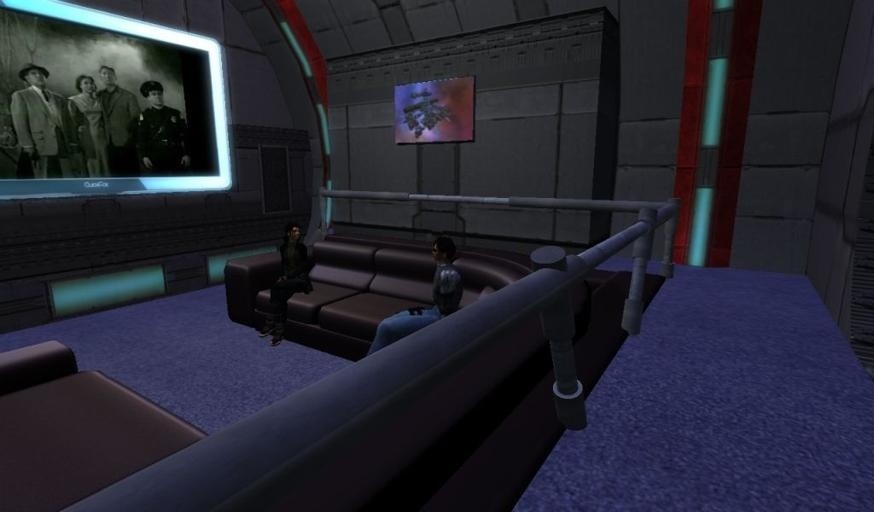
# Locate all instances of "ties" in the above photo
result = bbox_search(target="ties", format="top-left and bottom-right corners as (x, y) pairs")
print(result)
(42, 89), (49, 101)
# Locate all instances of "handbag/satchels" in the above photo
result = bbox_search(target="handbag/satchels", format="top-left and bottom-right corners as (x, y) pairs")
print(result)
(144, 134), (165, 163)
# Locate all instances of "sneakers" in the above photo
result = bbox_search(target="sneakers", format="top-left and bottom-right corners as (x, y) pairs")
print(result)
(258, 321), (284, 346)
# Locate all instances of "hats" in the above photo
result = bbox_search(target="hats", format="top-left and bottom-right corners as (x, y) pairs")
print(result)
(140, 80), (163, 96)
(18, 63), (49, 80)
